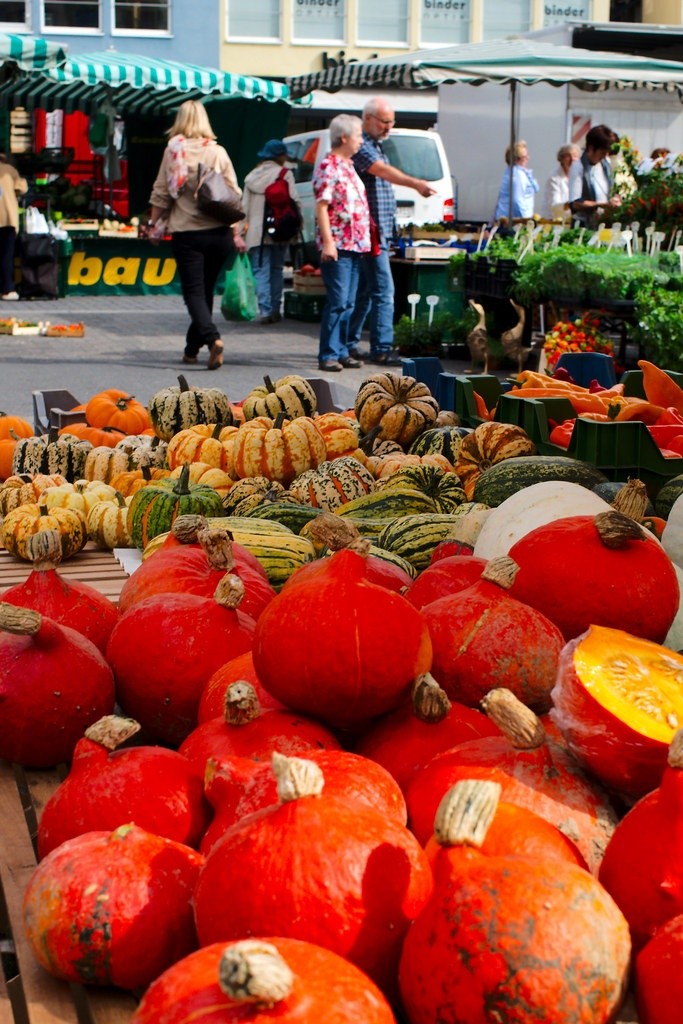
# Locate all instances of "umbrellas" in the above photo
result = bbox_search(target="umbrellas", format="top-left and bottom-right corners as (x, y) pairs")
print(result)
(0, 31), (317, 219)
(287, 35), (682, 237)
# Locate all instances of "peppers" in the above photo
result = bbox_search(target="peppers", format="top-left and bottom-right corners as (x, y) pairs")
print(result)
(473, 359), (683, 458)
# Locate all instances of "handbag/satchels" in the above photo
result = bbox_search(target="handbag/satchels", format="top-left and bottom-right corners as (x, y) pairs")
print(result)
(194, 138), (246, 223)
(368, 216), (382, 258)
(220, 251), (258, 321)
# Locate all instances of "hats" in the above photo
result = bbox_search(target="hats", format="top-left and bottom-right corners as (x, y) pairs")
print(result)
(257, 140), (287, 158)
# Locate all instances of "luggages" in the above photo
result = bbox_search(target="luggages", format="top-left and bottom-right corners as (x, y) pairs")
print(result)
(14, 195), (60, 300)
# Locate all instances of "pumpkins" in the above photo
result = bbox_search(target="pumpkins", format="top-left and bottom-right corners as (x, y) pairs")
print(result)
(299, 263), (321, 277)
(0, 454), (683, 1024)
(0, 372), (538, 547)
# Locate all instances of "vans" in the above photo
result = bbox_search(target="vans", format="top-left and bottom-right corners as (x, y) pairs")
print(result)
(279, 125), (455, 270)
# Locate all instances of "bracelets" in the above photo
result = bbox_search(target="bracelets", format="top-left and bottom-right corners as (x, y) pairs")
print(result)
(146, 223), (155, 228)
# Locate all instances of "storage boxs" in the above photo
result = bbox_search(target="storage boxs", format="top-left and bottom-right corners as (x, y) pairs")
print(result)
(283, 267), (326, 324)
(398, 352), (683, 504)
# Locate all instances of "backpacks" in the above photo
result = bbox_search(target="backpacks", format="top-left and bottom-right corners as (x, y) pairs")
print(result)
(262, 171), (301, 242)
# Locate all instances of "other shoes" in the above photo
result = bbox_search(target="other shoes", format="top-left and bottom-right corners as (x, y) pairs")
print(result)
(0, 291), (21, 301)
(182, 354), (197, 364)
(208, 336), (224, 369)
(371, 351), (406, 366)
(339, 354), (363, 367)
(261, 311), (280, 324)
(319, 359), (342, 373)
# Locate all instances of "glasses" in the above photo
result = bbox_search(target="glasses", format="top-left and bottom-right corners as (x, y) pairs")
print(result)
(369, 112), (396, 127)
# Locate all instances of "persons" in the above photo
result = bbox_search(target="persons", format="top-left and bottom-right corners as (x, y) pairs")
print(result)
(493, 140), (540, 222)
(351, 99), (438, 367)
(313, 113), (371, 371)
(148, 100), (248, 370)
(637, 147), (678, 177)
(0, 152), (29, 300)
(241, 138), (304, 326)
(546, 124), (640, 231)
